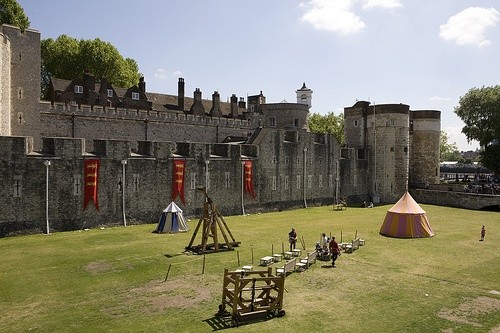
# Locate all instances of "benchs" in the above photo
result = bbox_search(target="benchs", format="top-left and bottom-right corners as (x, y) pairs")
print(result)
(299, 249), (319, 270)
(344, 237), (361, 254)
(260, 256), (274, 266)
(274, 257), (299, 280)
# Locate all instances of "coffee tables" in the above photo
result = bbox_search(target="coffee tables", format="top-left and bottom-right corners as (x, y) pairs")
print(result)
(360, 240), (365, 246)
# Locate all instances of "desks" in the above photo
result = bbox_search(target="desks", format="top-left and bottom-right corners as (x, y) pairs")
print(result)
(342, 242), (349, 250)
(296, 262), (306, 272)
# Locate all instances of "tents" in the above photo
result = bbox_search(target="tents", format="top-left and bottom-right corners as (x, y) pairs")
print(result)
(380, 191), (435, 238)
(155, 199), (190, 234)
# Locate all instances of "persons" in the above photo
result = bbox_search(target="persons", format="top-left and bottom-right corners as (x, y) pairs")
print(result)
(480, 225), (486, 241)
(289, 228), (297, 252)
(316, 233), (328, 253)
(361, 200), (373, 208)
(329, 237), (340, 267)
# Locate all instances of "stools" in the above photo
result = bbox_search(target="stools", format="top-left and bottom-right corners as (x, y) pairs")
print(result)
(284, 251), (292, 259)
(242, 265), (254, 271)
(292, 249), (302, 257)
(273, 253), (283, 262)
